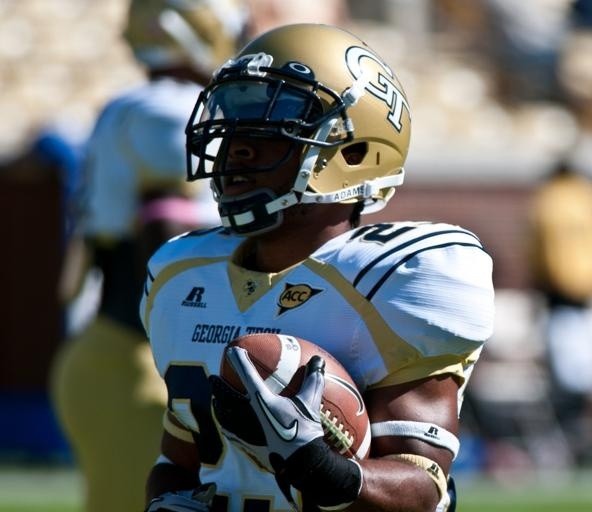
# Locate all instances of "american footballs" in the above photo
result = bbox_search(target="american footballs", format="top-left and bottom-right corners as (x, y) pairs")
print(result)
(221, 332), (372, 461)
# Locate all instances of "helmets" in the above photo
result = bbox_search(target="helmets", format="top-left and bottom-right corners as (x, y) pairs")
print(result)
(128, 0), (250, 84)
(185, 23), (411, 236)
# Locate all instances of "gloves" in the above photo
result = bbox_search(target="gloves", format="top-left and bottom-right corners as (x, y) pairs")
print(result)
(207, 346), (363, 512)
(145, 483), (216, 512)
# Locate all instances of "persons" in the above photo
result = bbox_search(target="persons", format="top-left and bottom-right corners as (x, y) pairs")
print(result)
(527, 34), (591, 476)
(134, 22), (499, 511)
(47, 1), (254, 510)
(1, 0), (588, 186)
